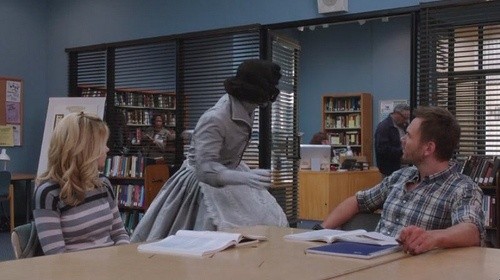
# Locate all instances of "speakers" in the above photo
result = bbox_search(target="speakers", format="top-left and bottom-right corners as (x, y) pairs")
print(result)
(318, 0), (348, 14)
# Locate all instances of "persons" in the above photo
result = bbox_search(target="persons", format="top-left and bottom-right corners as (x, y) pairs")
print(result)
(31, 112), (130, 256)
(312, 108), (485, 256)
(374, 104), (411, 182)
(129, 58), (287, 243)
(140, 113), (171, 145)
(311, 132), (329, 146)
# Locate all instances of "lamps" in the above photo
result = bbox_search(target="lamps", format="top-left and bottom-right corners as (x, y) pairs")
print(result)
(0, 148), (11, 171)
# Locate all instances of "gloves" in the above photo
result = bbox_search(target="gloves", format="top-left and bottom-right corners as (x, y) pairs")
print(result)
(216, 168), (272, 191)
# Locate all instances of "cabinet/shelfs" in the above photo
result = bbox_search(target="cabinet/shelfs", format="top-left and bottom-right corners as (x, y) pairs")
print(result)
(100, 157), (169, 235)
(79, 86), (176, 148)
(454, 153), (500, 250)
(296, 164), (383, 228)
(321, 93), (373, 167)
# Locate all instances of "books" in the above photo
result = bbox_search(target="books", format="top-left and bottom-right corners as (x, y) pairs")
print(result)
(105, 155), (164, 229)
(325, 97), (361, 145)
(285, 229), (400, 246)
(81, 88), (176, 144)
(138, 230), (267, 259)
(456, 156), (500, 229)
(304, 241), (402, 260)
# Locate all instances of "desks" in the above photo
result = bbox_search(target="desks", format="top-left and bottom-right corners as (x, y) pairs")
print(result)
(10, 173), (37, 225)
(0, 225), (500, 280)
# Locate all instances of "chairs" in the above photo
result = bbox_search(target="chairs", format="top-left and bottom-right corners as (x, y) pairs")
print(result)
(0, 171), (15, 233)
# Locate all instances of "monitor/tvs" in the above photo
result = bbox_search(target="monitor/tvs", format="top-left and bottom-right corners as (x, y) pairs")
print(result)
(300, 144), (331, 171)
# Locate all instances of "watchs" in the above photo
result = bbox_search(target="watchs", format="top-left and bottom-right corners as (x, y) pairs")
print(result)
(312, 223), (324, 231)
